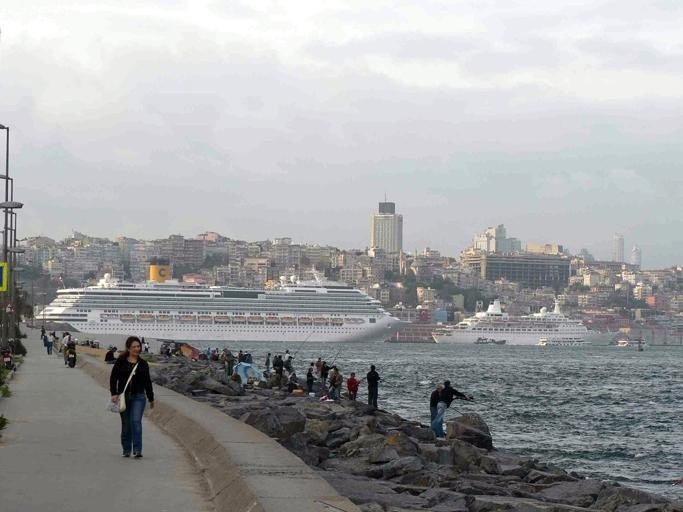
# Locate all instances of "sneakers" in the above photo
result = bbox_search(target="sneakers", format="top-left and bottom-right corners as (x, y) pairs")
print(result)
(132, 450), (142, 457)
(121, 449), (131, 457)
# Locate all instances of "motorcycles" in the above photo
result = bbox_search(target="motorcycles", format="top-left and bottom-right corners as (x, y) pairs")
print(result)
(68, 350), (75, 368)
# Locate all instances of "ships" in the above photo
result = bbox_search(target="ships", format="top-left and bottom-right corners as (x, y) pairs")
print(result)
(34, 270), (411, 342)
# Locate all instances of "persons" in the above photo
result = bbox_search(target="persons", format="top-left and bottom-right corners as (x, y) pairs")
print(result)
(40, 325), (75, 365)
(367, 365), (380, 408)
(141, 337), (146, 352)
(159, 340), (360, 401)
(428, 380), (471, 441)
(105, 347), (118, 364)
(144, 342), (150, 352)
(430, 383), (445, 426)
(110, 335), (155, 458)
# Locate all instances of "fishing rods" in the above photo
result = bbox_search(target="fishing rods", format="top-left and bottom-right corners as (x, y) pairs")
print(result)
(292, 323), (324, 360)
(329, 346), (342, 369)
(449, 396), (507, 416)
(378, 377), (398, 388)
(357, 354), (403, 384)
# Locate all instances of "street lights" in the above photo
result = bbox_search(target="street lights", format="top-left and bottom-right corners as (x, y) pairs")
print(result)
(1, 124), (25, 306)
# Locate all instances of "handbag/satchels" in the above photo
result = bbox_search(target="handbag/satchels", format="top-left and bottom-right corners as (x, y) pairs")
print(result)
(105, 393), (125, 413)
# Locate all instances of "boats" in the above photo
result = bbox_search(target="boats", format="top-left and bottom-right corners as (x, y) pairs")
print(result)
(446, 414), (492, 445)
(617, 340), (648, 347)
(431, 299), (597, 347)
